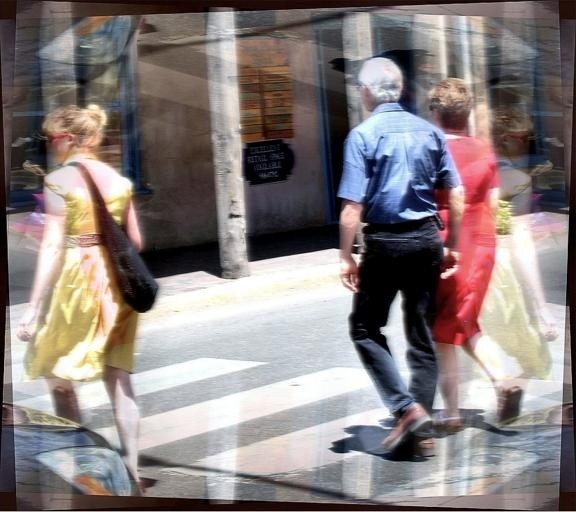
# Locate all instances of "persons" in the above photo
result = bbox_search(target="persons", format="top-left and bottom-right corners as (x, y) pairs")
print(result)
(476, 102), (563, 347)
(13, 102), (157, 490)
(337, 58), (465, 457)
(424, 78), (524, 431)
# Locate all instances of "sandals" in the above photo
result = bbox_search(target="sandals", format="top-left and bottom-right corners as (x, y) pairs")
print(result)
(431, 410), (464, 432)
(497, 382), (522, 425)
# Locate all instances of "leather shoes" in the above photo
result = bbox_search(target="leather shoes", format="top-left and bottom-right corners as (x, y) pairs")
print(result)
(414, 434), (436, 457)
(381, 402), (433, 451)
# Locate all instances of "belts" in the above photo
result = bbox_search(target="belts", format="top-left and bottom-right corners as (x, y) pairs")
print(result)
(362, 215), (440, 235)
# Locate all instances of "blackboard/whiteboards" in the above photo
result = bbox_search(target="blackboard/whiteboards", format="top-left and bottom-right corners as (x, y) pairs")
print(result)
(244, 140), (296, 186)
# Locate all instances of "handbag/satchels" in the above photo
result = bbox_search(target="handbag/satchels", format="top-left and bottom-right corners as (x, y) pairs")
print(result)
(65, 162), (159, 314)
